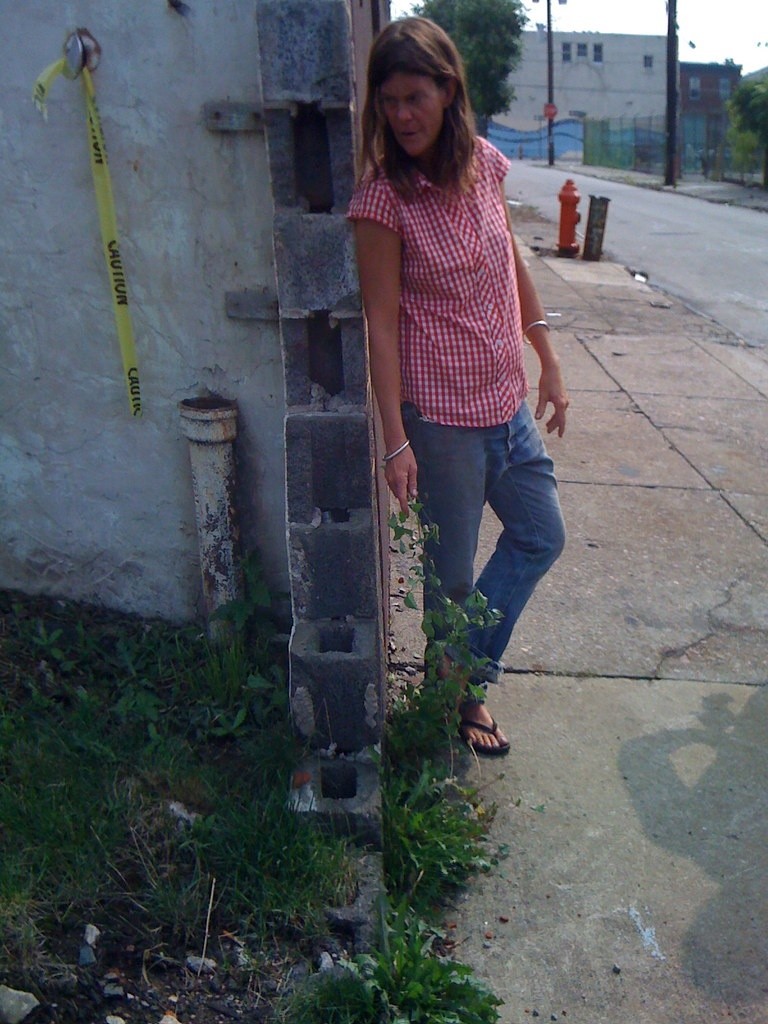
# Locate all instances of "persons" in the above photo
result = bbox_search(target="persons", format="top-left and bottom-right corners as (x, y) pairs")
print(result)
(344, 16), (570, 756)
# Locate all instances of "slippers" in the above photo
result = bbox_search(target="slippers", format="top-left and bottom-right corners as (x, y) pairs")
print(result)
(454, 703), (511, 754)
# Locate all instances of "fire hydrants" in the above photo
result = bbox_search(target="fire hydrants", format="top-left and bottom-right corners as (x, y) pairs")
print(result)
(556, 179), (581, 258)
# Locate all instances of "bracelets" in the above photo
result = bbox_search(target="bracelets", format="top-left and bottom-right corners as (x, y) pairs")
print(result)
(382, 439), (410, 461)
(523, 320), (550, 345)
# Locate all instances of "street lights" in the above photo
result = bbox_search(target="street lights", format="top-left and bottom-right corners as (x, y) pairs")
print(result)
(532, 0), (567, 166)
